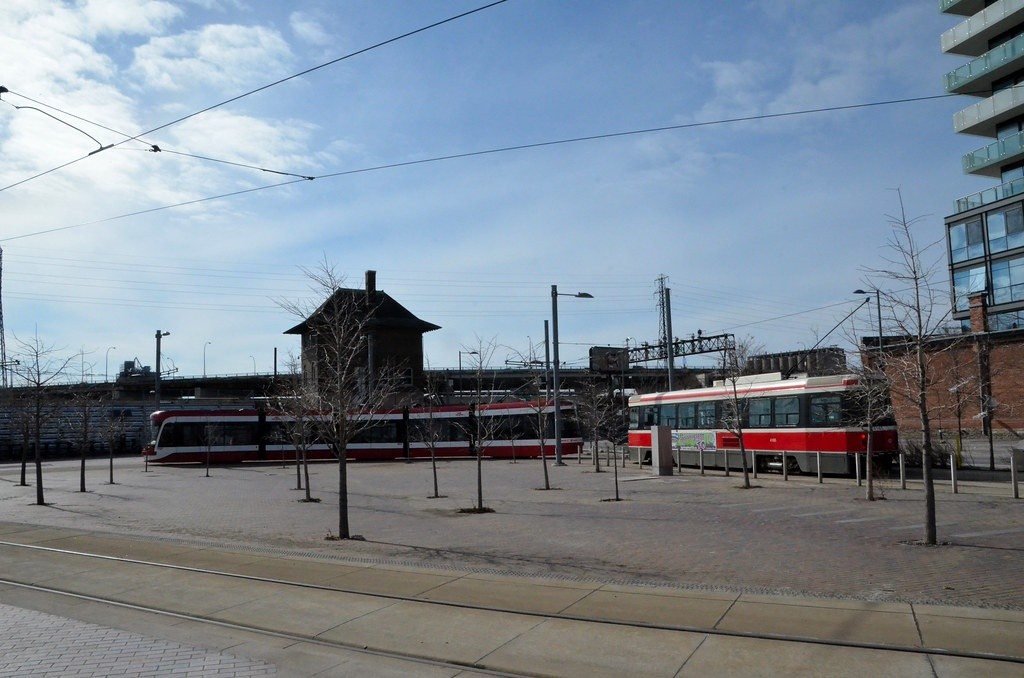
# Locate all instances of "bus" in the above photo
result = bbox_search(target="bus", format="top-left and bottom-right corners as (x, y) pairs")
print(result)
(627, 372), (899, 479)
(141, 388), (585, 466)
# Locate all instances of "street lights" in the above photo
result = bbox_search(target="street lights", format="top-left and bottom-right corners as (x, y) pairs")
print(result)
(203, 341), (212, 376)
(153, 329), (171, 409)
(459, 350), (478, 398)
(250, 356), (256, 376)
(852, 289), (883, 367)
(105, 346), (116, 383)
(550, 284), (595, 467)
